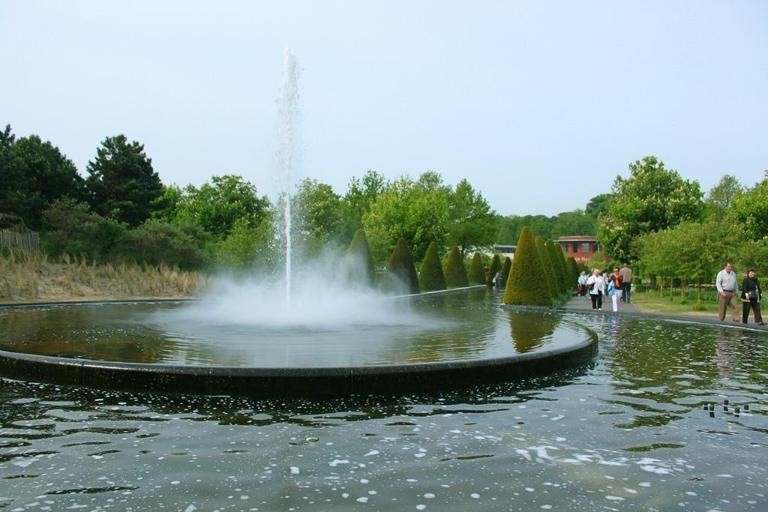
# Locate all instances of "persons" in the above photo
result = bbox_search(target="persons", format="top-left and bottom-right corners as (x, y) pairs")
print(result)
(578, 262), (634, 314)
(716, 262), (740, 323)
(740, 269), (764, 325)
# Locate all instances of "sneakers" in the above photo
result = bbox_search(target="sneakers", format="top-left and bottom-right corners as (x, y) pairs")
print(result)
(594, 308), (596, 310)
(599, 307), (602, 310)
(756, 321), (763, 325)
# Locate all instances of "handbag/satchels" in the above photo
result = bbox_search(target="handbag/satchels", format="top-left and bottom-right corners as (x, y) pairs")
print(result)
(588, 283), (594, 289)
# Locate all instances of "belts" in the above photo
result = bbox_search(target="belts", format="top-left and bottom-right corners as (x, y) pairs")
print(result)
(723, 289), (734, 292)
